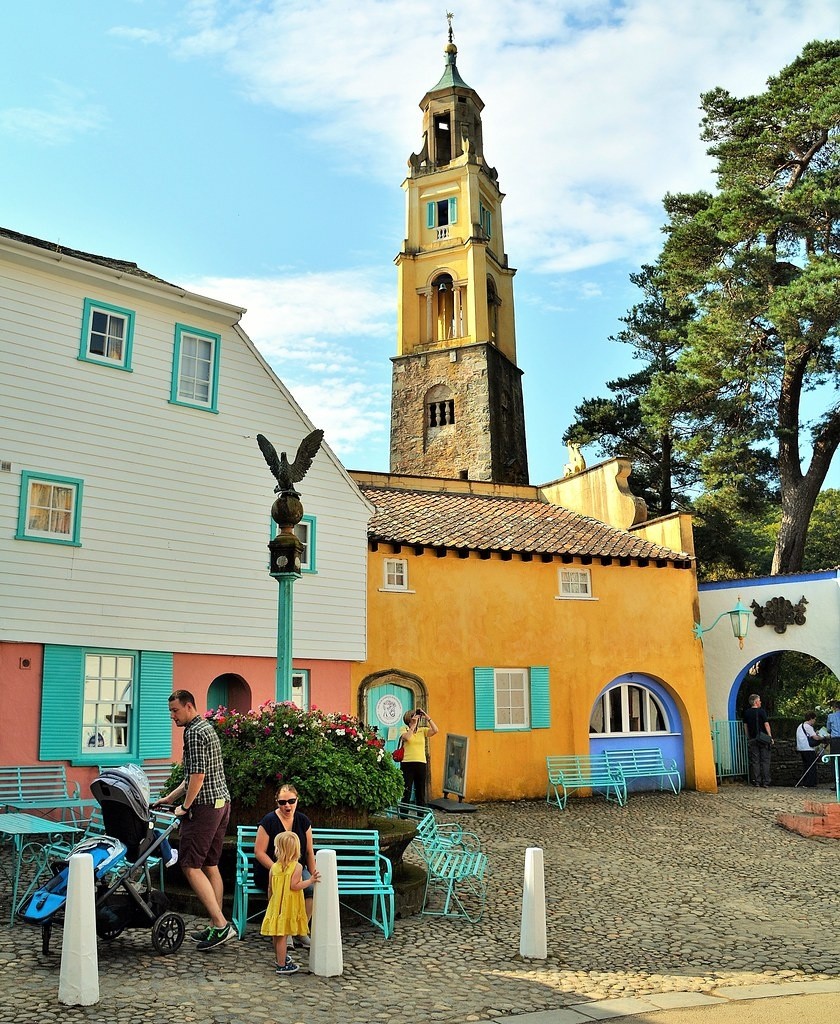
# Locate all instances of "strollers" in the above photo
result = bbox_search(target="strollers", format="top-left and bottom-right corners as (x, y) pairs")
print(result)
(17, 770), (186, 954)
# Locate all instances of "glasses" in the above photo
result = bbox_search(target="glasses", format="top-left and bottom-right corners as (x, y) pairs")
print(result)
(276, 797), (297, 805)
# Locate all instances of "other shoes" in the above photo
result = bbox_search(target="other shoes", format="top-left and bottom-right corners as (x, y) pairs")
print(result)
(763, 782), (770, 788)
(803, 785), (818, 788)
(754, 782), (760, 787)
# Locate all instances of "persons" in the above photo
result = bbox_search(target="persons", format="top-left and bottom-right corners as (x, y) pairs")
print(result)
(260, 831), (321, 973)
(254, 784), (315, 950)
(743, 694), (775, 788)
(152, 689), (237, 951)
(399, 708), (438, 827)
(796, 701), (840, 790)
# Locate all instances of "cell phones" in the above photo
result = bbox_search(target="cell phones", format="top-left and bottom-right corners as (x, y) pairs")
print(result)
(416, 709), (420, 715)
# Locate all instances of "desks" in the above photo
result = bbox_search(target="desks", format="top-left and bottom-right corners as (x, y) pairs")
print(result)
(0, 813), (86, 928)
(4, 799), (98, 849)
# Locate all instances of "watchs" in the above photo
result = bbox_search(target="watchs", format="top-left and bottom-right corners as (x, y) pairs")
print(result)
(180, 804), (190, 812)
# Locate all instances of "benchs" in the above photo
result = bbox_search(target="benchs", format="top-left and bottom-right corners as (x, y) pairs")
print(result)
(545, 756), (626, 809)
(97, 762), (183, 799)
(379, 803), (468, 880)
(40, 802), (179, 889)
(232, 825), (395, 942)
(603, 747), (680, 804)
(418, 813), (492, 924)
(0, 764), (83, 833)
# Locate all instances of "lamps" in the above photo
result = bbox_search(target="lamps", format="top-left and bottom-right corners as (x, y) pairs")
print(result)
(692, 592), (753, 649)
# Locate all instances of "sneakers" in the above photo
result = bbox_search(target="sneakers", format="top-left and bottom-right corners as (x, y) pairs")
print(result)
(286, 935), (294, 948)
(191, 926), (214, 941)
(293, 935), (311, 947)
(275, 956), (292, 967)
(196, 923), (236, 950)
(276, 964), (299, 973)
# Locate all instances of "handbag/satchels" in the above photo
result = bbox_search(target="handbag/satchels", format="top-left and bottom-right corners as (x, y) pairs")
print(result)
(808, 736), (821, 747)
(393, 736), (406, 762)
(755, 732), (772, 747)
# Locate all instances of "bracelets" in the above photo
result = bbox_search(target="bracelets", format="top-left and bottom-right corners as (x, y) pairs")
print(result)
(428, 718), (431, 722)
